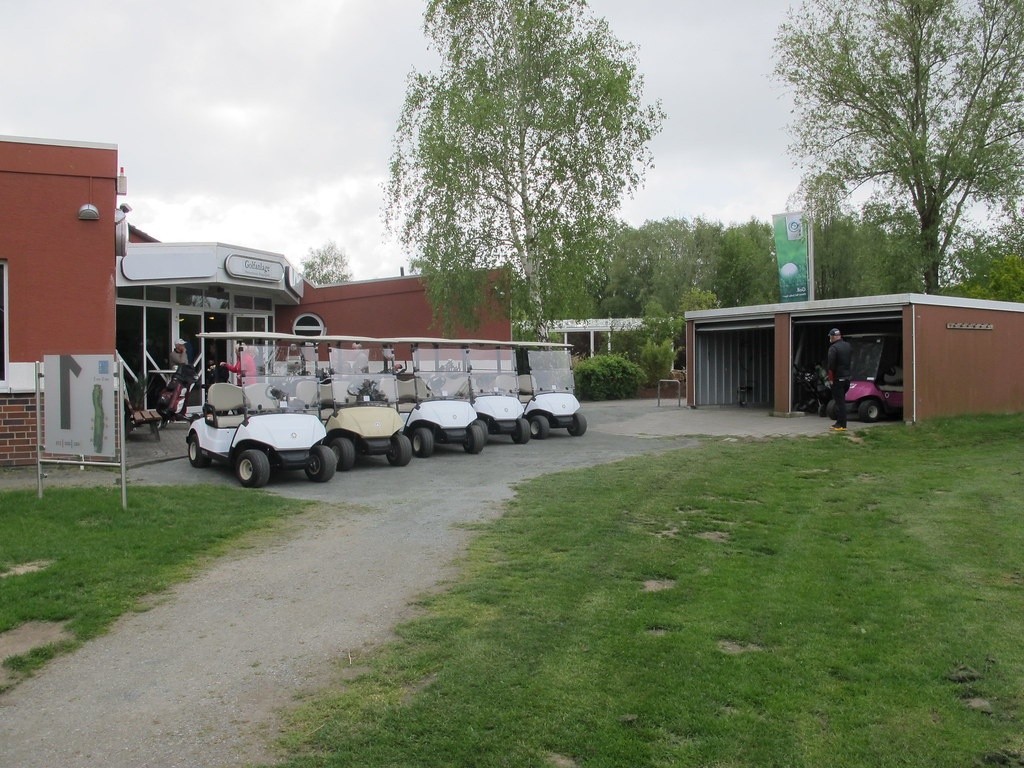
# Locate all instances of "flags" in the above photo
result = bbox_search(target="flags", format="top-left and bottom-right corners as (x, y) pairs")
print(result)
(770, 211), (809, 302)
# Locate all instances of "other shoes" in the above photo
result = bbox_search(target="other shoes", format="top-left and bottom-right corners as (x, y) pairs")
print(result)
(830, 422), (848, 431)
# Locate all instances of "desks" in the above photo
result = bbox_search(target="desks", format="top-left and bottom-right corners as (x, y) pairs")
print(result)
(148, 369), (177, 389)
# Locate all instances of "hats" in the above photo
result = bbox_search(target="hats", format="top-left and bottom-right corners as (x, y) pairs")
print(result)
(174, 339), (188, 344)
(827, 329), (841, 336)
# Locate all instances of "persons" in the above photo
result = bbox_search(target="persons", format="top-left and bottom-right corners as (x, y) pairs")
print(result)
(219, 342), (255, 387)
(826, 327), (851, 432)
(290, 342), (307, 376)
(171, 339), (189, 373)
(351, 343), (369, 374)
(247, 341), (265, 383)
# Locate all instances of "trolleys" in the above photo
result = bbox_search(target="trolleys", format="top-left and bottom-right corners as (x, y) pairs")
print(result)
(792, 363), (827, 417)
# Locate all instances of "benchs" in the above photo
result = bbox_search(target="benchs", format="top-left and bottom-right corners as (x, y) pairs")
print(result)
(124, 398), (161, 442)
(429, 376), (477, 400)
(496, 375), (537, 403)
(377, 377), (427, 413)
(297, 379), (356, 420)
(208, 383), (282, 429)
